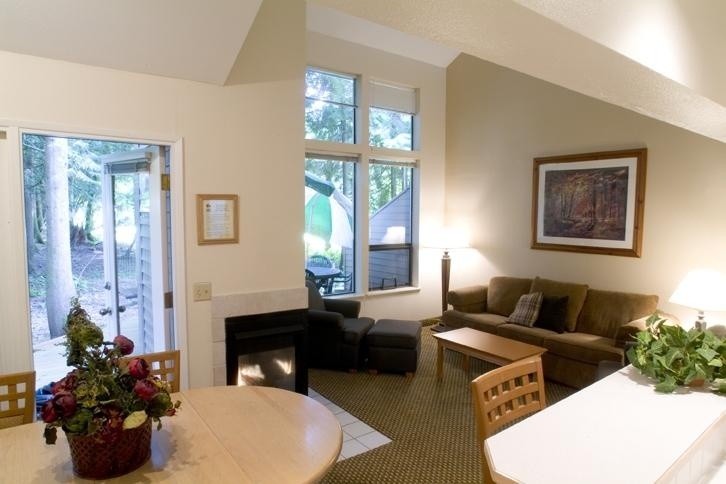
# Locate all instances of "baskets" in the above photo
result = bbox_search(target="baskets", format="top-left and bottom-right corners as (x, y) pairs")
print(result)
(60, 414), (152, 480)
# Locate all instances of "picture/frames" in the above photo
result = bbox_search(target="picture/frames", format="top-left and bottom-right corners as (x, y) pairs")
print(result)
(194, 193), (240, 245)
(530, 146), (647, 258)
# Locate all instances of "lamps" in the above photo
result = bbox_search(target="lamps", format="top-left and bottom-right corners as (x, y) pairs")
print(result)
(420, 223), (477, 312)
(665, 264), (726, 334)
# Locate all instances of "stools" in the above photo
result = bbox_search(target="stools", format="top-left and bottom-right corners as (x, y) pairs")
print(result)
(363, 316), (423, 380)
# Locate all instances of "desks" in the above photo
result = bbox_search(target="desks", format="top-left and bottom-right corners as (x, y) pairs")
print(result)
(0, 384), (344, 484)
(483, 360), (726, 483)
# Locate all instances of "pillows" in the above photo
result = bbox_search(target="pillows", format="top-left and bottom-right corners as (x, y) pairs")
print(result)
(528, 274), (589, 335)
(535, 291), (571, 334)
(505, 290), (545, 326)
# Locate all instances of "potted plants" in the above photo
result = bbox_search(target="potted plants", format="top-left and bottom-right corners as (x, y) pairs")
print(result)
(621, 307), (726, 395)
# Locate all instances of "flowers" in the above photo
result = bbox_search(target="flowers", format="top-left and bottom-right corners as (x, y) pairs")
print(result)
(40, 296), (182, 448)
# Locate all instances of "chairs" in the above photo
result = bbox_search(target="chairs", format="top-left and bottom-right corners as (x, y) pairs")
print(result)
(109, 345), (180, 395)
(469, 351), (558, 483)
(302, 268), (377, 377)
(0, 367), (38, 429)
(304, 254), (355, 298)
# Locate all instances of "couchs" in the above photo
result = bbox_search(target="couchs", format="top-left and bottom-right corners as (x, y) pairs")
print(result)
(439, 272), (663, 392)
